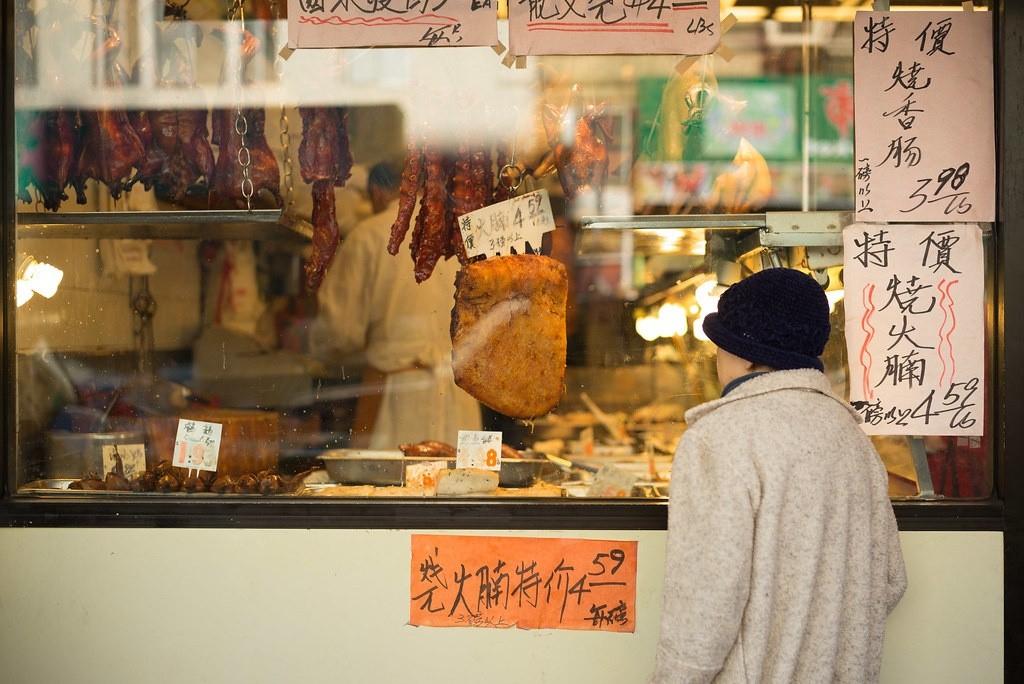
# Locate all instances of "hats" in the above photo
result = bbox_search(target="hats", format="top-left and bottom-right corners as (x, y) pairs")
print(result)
(702, 266), (833, 370)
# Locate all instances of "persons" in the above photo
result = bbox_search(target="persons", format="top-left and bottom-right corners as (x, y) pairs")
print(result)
(283, 161), (482, 452)
(650, 268), (907, 684)
